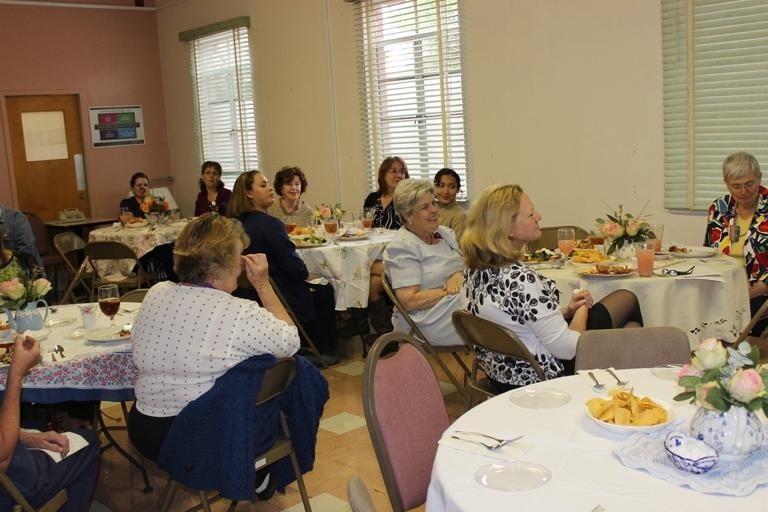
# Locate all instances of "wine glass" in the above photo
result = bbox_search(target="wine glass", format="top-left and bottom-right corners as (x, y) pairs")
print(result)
(278, 206), (381, 247)
(97, 281), (127, 338)
(556, 227), (576, 271)
(118, 207), (129, 231)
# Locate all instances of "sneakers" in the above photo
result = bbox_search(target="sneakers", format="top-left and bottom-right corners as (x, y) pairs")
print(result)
(362, 341), (399, 358)
(302, 353), (340, 366)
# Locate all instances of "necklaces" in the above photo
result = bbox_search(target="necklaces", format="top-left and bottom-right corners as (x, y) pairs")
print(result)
(426, 233), (435, 246)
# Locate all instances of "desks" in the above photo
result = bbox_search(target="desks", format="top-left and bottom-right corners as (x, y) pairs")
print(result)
(289, 221), (399, 356)
(42, 212), (202, 288)
(4, 302), (156, 496)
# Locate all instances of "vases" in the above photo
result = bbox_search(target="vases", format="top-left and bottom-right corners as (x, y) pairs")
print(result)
(690, 407), (764, 461)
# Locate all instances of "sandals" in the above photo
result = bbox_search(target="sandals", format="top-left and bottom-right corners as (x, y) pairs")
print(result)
(253, 464), (278, 501)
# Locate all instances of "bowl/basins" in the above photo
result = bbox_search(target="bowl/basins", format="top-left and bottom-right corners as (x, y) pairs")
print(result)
(664, 431), (719, 475)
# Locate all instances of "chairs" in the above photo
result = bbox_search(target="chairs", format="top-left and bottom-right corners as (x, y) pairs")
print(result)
(452, 309), (547, 410)
(381, 270), (471, 402)
(157, 356), (315, 511)
(21, 210), (150, 302)
(526, 226), (590, 252)
(0, 470), (70, 512)
(574, 326), (691, 374)
(234, 269), (330, 369)
(731, 299), (768, 350)
(362, 331), (451, 512)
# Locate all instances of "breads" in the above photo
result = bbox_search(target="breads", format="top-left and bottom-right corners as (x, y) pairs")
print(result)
(129, 217), (144, 223)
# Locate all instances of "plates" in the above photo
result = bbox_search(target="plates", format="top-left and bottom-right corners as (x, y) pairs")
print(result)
(507, 386), (571, 410)
(581, 265), (633, 278)
(585, 397), (680, 436)
(0, 338), (19, 369)
(85, 321), (131, 348)
(473, 462), (551, 493)
(654, 253), (669, 259)
(666, 246), (713, 260)
(128, 223), (148, 228)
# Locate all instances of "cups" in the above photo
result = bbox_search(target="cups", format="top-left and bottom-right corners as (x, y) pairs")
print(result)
(634, 243), (655, 278)
(77, 304), (101, 326)
(646, 222), (662, 251)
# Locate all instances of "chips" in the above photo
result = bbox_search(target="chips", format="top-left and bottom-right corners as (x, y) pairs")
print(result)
(291, 227), (313, 234)
(587, 388), (668, 426)
(573, 251), (610, 262)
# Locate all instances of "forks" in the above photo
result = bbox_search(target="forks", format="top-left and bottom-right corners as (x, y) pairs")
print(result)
(449, 429), (527, 451)
(48, 347), (57, 362)
(603, 366), (631, 389)
(588, 373), (604, 394)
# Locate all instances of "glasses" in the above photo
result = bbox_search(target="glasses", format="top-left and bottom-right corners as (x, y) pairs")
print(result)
(662, 266), (695, 276)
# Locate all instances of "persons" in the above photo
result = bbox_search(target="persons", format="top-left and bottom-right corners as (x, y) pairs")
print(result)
(118, 171), (179, 283)
(0, 332), (101, 510)
(127, 213), (303, 501)
(362, 155), (411, 232)
(226, 169), (340, 366)
(381, 178), (471, 345)
(0, 205), (47, 283)
(456, 185), (647, 387)
(337, 168), (470, 359)
(701, 150), (768, 340)
(265, 165), (317, 229)
(194, 160), (234, 217)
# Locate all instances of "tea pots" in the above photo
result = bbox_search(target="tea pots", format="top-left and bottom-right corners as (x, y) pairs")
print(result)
(144, 212), (164, 226)
(2, 303), (48, 334)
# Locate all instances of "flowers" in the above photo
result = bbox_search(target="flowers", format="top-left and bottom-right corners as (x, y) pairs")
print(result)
(315, 203), (346, 220)
(595, 197), (657, 255)
(139, 196), (169, 213)
(673, 337), (768, 412)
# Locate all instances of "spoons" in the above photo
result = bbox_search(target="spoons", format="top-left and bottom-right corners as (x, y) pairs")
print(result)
(54, 344), (65, 358)
